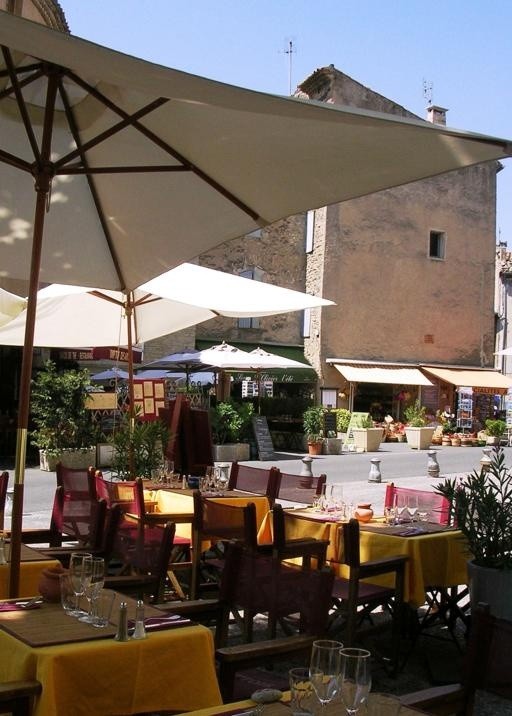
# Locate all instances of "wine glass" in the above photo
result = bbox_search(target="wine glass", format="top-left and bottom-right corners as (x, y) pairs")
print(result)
(77, 556), (104, 625)
(405, 492), (417, 531)
(149, 460), (233, 498)
(392, 493), (408, 526)
(312, 482), (355, 523)
(66, 551), (90, 618)
(336, 647), (372, 715)
(307, 640), (346, 715)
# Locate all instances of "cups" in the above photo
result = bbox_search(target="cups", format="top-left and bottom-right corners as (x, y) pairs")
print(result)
(59, 572), (84, 615)
(385, 506), (396, 526)
(288, 667), (324, 714)
(89, 588), (115, 629)
(417, 511), (434, 531)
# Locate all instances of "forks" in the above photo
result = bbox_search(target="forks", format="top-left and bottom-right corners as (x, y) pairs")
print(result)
(0, 596), (44, 611)
(125, 614), (182, 629)
(232, 704), (265, 716)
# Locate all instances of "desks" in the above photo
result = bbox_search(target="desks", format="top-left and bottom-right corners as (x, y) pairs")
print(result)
(177, 671), (437, 714)
(0, 588), (224, 716)
(107, 475), (274, 586)
(278, 501), (463, 656)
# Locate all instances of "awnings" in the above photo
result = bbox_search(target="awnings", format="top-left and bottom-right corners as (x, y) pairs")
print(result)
(419, 363), (512, 388)
(325, 358), (434, 386)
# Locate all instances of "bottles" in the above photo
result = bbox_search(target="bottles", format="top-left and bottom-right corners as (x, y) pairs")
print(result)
(113, 599), (130, 643)
(132, 600), (147, 640)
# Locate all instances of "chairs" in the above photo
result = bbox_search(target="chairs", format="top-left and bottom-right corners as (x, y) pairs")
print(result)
(385, 481), (511, 615)
(1, 460), (176, 604)
(192, 486), (414, 656)
(266, 416), (306, 452)
(228, 458), (328, 508)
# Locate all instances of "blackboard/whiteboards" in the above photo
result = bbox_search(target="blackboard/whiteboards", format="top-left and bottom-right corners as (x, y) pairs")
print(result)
(324, 412), (336, 432)
(251, 414), (275, 460)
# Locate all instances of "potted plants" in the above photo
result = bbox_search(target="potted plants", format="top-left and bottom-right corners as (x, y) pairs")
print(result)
(300, 405), (324, 456)
(211, 400), (257, 465)
(323, 430), (343, 456)
(380, 395), (507, 450)
(25, 358), (96, 471)
(428, 439), (512, 625)
(351, 419), (383, 452)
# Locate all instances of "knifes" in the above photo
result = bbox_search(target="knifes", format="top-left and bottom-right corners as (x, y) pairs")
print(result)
(127, 618), (192, 629)
